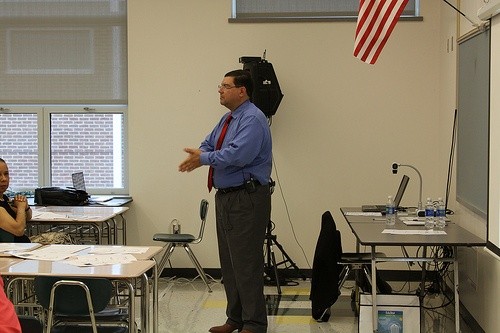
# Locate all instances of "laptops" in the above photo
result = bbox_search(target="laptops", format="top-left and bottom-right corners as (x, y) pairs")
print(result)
(72, 172), (113, 202)
(362, 175), (410, 212)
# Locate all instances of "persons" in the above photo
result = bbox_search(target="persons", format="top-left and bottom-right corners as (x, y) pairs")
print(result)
(178, 69), (272, 333)
(0, 158), (32, 243)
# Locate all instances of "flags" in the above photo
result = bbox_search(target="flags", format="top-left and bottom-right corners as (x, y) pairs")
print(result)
(353, 0), (410, 64)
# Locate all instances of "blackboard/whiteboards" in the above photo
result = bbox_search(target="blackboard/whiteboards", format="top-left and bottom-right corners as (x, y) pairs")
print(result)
(455, 20), (490, 220)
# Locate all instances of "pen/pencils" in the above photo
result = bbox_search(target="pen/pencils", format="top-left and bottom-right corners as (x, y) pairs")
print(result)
(373, 218), (386, 221)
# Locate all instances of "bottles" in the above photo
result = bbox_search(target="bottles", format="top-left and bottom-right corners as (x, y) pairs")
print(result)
(435, 198), (445, 228)
(386, 196), (395, 225)
(425, 197), (434, 228)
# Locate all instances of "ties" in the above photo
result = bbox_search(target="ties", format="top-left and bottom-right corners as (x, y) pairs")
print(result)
(208, 114), (233, 193)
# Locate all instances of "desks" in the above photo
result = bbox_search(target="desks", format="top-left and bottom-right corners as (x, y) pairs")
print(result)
(340, 207), (487, 333)
(0, 198), (163, 333)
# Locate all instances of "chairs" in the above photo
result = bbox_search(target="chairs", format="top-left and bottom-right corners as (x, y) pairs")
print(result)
(310, 211), (386, 322)
(33, 276), (129, 333)
(150, 200), (212, 293)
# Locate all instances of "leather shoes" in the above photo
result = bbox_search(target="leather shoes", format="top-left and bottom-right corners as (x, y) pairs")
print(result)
(239, 329), (252, 333)
(209, 322), (238, 333)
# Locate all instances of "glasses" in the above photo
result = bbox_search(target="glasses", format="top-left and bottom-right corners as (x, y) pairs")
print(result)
(217, 84), (241, 89)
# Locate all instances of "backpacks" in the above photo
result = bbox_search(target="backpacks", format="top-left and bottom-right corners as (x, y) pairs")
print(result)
(243, 59), (285, 127)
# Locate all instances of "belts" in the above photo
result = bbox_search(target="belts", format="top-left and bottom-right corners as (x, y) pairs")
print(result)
(218, 181), (259, 194)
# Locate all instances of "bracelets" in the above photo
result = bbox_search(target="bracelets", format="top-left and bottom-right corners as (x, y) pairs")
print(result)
(25, 207), (30, 211)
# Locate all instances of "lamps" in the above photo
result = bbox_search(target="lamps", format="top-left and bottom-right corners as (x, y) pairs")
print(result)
(392, 163), (425, 217)
